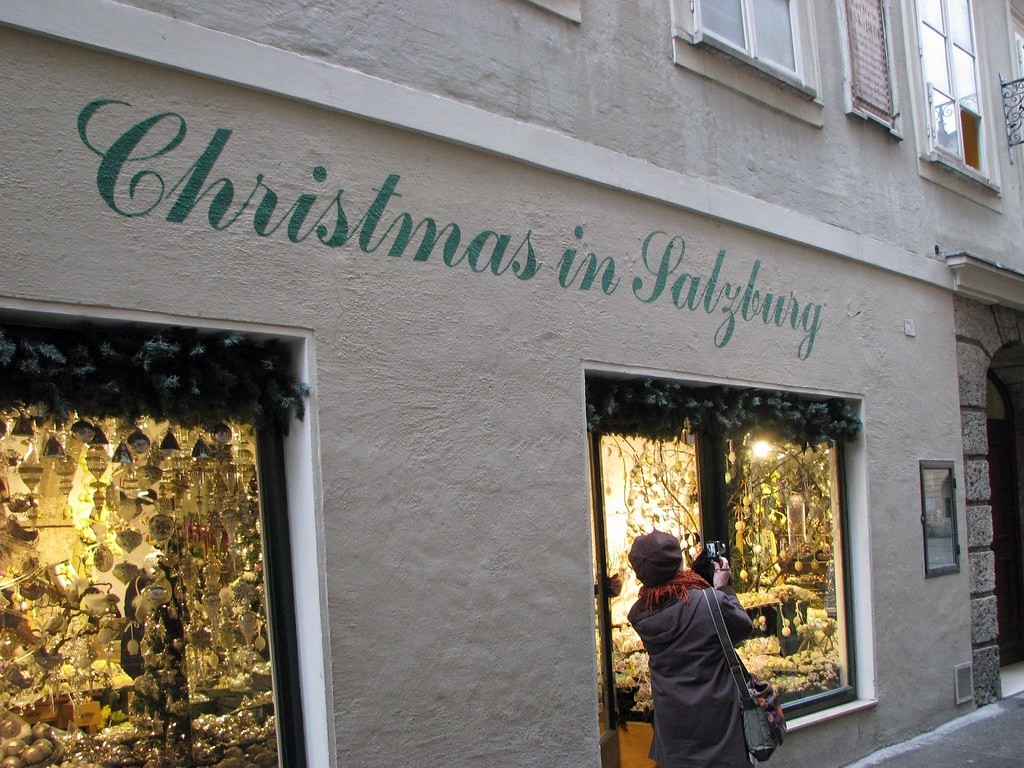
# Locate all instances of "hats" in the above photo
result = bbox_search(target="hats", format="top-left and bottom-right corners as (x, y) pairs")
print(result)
(628, 529), (683, 588)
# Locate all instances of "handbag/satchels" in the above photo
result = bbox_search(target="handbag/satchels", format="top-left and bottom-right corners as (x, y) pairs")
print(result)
(702, 586), (788, 762)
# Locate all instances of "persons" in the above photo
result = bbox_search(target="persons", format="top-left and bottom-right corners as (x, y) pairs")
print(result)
(121, 521), (192, 768)
(627, 530), (754, 768)
(594, 573), (622, 597)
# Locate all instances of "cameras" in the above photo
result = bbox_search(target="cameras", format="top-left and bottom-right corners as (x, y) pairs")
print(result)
(706, 541), (726, 565)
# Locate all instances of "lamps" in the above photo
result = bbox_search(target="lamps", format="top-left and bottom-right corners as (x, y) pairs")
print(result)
(0, 433), (278, 768)
